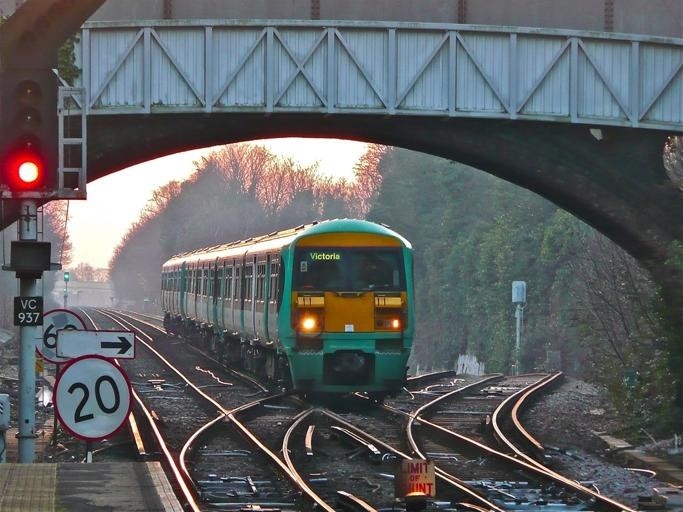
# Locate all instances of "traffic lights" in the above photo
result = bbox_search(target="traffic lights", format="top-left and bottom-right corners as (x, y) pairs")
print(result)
(5, 41), (65, 195)
(64, 271), (70, 284)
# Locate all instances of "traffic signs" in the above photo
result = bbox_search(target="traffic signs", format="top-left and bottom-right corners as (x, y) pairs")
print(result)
(36, 310), (87, 364)
(53, 354), (132, 441)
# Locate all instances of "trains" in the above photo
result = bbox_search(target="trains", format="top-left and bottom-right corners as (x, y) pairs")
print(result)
(159, 216), (419, 403)
